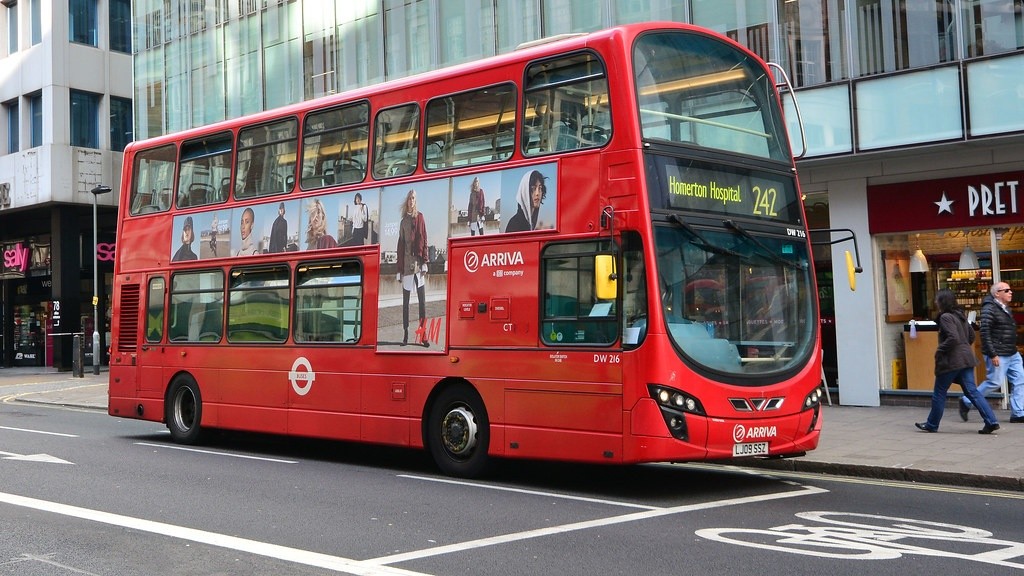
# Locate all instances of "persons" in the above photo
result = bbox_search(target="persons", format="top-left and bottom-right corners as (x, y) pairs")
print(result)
(173, 170), (552, 345)
(958, 282), (1024, 423)
(914, 288), (1000, 435)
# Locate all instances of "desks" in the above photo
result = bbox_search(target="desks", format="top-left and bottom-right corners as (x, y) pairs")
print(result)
(904, 331), (987, 391)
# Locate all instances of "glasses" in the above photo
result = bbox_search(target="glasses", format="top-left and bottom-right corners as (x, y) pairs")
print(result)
(998, 289), (1011, 292)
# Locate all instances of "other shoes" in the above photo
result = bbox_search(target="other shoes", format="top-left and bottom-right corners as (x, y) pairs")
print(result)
(1010, 416), (1024, 422)
(915, 423), (937, 432)
(979, 423), (999, 433)
(959, 399), (970, 421)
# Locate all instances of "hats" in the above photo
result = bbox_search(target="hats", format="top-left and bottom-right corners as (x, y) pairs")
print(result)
(183, 216), (193, 229)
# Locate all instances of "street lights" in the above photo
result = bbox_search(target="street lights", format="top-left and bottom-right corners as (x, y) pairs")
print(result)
(90, 184), (112, 376)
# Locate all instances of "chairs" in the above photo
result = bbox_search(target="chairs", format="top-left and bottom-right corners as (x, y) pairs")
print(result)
(153, 157), (365, 210)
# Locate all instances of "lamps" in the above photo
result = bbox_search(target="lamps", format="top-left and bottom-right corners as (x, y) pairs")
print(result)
(908, 235), (929, 273)
(959, 232), (979, 269)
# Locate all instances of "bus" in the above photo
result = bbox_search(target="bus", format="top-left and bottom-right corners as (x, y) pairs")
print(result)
(106, 22), (864, 478)
